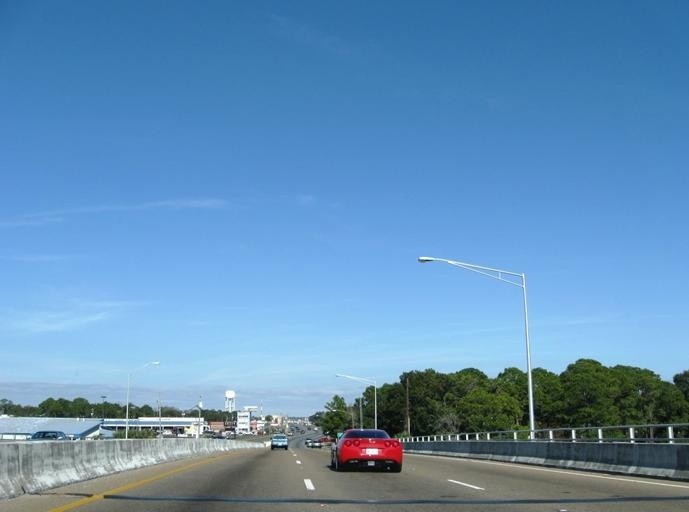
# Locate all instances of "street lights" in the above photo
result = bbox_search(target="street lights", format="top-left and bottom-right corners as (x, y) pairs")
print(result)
(125, 360), (160, 439)
(419, 256), (536, 441)
(336, 372), (378, 429)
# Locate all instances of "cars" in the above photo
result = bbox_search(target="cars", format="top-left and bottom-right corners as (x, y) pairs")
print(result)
(31, 430), (68, 440)
(305, 437), (322, 449)
(331, 428), (403, 471)
(271, 433), (289, 450)
